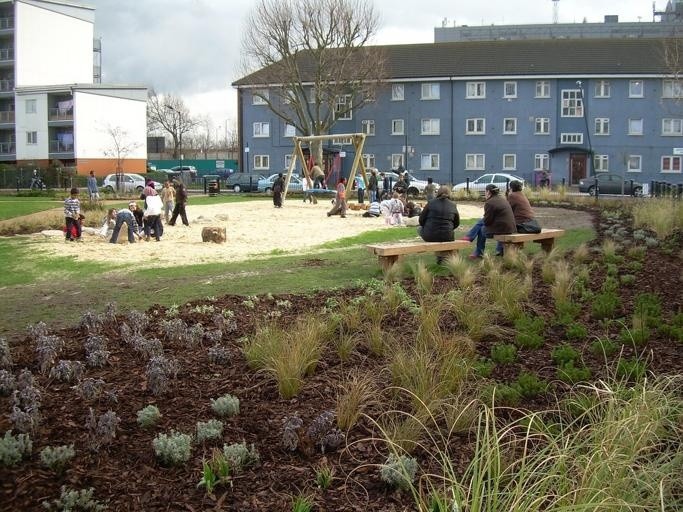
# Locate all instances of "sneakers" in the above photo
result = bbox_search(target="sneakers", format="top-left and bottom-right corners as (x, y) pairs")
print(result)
(468, 253), (485, 263)
(64, 237), (71, 244)
(491, 250), (504, 257)
(75, 236), (85, 243)
(455, 236), (473, 243)
(138, 233), (147, 241)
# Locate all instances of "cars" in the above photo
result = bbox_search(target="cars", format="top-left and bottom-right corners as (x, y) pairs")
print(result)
(102, 173), (164, 193)
(225, 173), (267, 194)
(257, 173), (303, 196)
(579, 172), (643, 197)
(452, 173), (526, 195)
(147, 163), (233, 182)
(353, 170), (440, 199)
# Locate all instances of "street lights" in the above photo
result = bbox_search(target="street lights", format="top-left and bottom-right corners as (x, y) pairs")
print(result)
(166, 105), (183, 176)
(575, 80), (600, 195)
(244, 141), (250, 174)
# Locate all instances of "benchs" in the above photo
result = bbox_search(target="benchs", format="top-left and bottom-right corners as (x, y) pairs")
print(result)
(365, 237), (470, 277)
(492, 227), (566, 262)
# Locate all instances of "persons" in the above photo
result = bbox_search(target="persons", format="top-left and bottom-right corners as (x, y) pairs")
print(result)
(541, 168), (550, 189)
(64, 187), (82, 242)
(64, 214), (85, 242)
(39, 179), (44, 184)
(100, 175), (188, 244)
(88, 170), (100, 206)
(496, 179), (536, 255)
(271, 172), (284, 208)
(460, 184), (516, 262)
(303, 162), (436, 225)
(283, 176), (285, 181)
(30, 169), (38, 188)
(421, 186), (461, 264)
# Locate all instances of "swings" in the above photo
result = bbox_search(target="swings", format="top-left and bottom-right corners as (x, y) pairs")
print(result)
(303, 140), (338, 194)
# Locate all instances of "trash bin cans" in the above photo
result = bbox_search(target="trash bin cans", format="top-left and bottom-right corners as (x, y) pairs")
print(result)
(208, 180), (218, 196)
